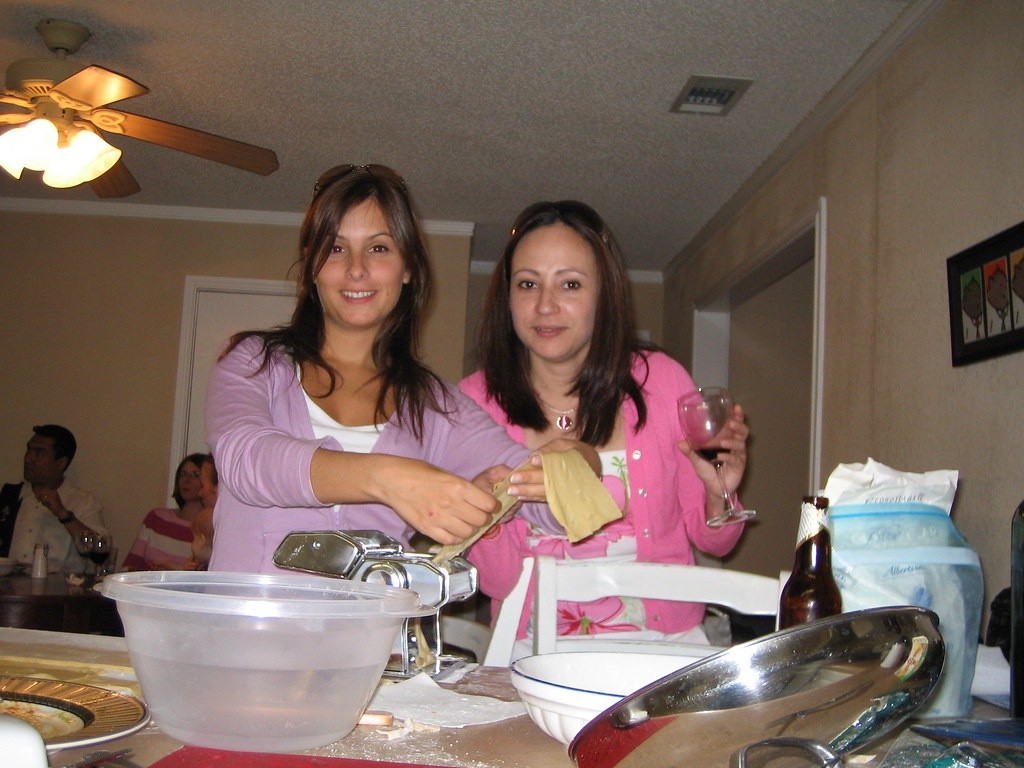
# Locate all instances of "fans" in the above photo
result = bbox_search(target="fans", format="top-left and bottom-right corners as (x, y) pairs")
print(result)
(0, 15), (283, 200)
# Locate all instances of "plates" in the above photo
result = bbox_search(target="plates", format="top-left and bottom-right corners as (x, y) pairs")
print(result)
(0, 674), (151, 756)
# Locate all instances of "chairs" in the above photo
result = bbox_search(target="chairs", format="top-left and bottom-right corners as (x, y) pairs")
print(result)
(439, 554), (537, 667)
(527, 555), (793, 660)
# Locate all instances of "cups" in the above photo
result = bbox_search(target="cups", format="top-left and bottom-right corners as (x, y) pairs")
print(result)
(101, 547), (118, 575)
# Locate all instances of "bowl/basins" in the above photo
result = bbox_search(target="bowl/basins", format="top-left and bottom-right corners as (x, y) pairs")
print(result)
(509, 653), (710, 749)
(93, 569), (437, 758)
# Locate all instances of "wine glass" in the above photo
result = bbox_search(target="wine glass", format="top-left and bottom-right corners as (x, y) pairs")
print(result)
(76, 528), (95, 579)
(677, 385), (756, 527)
(87, 530), (112, 589)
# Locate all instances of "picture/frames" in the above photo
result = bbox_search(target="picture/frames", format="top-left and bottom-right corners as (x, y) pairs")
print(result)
(946, 219), (1024, 369)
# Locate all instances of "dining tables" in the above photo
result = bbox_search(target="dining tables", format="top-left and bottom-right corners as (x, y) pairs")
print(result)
(0, 573), (118, 634)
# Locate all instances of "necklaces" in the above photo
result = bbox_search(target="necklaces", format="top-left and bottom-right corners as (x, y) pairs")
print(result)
(536, 394), (578, 431)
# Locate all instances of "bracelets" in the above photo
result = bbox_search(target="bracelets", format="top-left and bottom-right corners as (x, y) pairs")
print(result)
(59, 512), (76, 525)
(54, 504), (64, 515)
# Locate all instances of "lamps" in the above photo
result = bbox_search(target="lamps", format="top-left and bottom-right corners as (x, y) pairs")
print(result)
(0, 110), (123, 191)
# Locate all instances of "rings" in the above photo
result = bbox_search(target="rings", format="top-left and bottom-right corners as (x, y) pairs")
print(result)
(44, 495), (48, 498)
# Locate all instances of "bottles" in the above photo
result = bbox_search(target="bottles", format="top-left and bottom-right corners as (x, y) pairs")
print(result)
(31, 542), (49, 579)
(778, 495), (842, 631)
(1010, 500), (1024, 719)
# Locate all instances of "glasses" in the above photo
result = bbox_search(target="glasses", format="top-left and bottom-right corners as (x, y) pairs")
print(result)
(177, 468), (201, 481)
(509, 200), (612, 255)
(312, 163), (409, 209)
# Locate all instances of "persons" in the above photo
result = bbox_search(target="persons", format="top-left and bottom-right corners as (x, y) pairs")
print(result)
(121, 451), (218, 573)
(445, 200), (750, 646)
(204, 162), (601, 601)
(0, 424), (113, 577)
